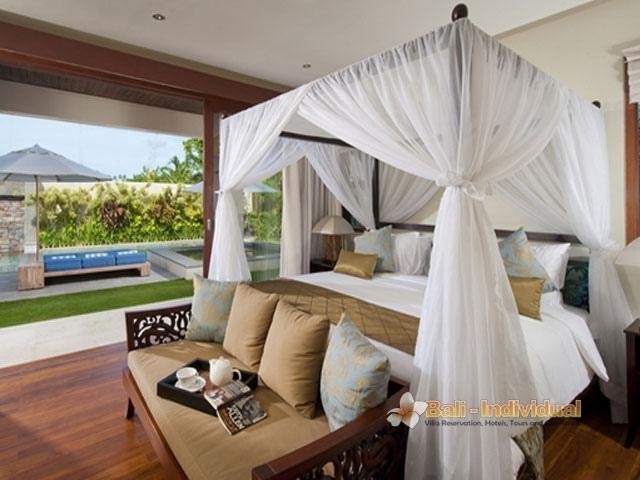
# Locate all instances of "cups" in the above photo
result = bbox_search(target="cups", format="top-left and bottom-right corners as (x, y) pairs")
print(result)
(176, 368), (197, 387)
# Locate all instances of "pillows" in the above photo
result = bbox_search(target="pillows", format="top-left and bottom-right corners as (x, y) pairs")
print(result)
(257, 298), (331, 420)
(388, 232), (433, 276)
(221, 282), (279, 373)
(351, 223), (397, 273)
(185, 273), (251, 342)
(494, 225), (556, 296)
(506, 273), (546, 323)
(319, 309), (391, 433)
(530, 238), (571, 293)
(332, 248), (380, 281)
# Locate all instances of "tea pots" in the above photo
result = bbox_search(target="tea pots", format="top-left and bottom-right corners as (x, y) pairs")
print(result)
(209, 357), (241, 387)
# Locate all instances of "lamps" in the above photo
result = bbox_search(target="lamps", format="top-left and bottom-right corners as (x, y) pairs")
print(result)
(312, 214), (355, 266)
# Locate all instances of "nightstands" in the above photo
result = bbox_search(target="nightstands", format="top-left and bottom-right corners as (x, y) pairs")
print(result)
(308, 258), (336, 273)
(623, 316), (640, 440)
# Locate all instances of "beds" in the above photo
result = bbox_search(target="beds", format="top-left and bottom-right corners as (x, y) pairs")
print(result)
(221, 242), (614, 479)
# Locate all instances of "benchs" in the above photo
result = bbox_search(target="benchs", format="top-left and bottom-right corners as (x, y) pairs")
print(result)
(19, 253), (152, 291)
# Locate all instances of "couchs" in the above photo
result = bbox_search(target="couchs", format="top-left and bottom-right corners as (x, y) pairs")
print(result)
(122, 302), (410, 480)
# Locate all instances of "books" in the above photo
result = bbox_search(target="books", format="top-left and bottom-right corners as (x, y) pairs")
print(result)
(203, 380), (270, 434)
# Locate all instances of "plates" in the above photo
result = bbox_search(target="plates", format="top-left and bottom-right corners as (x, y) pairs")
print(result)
(174, 377), (207, 391)
(156, 357), (259, 418)
